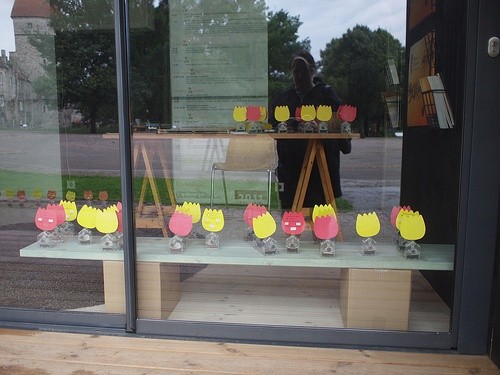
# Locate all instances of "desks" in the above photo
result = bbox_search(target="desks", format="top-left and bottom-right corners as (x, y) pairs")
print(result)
(101, 129), (360, 243)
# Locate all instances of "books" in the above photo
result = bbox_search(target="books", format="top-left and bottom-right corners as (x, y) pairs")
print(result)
(418, 73), (454, 130)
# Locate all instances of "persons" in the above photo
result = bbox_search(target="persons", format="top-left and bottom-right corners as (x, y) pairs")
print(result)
(268, 50), (354, 215)
